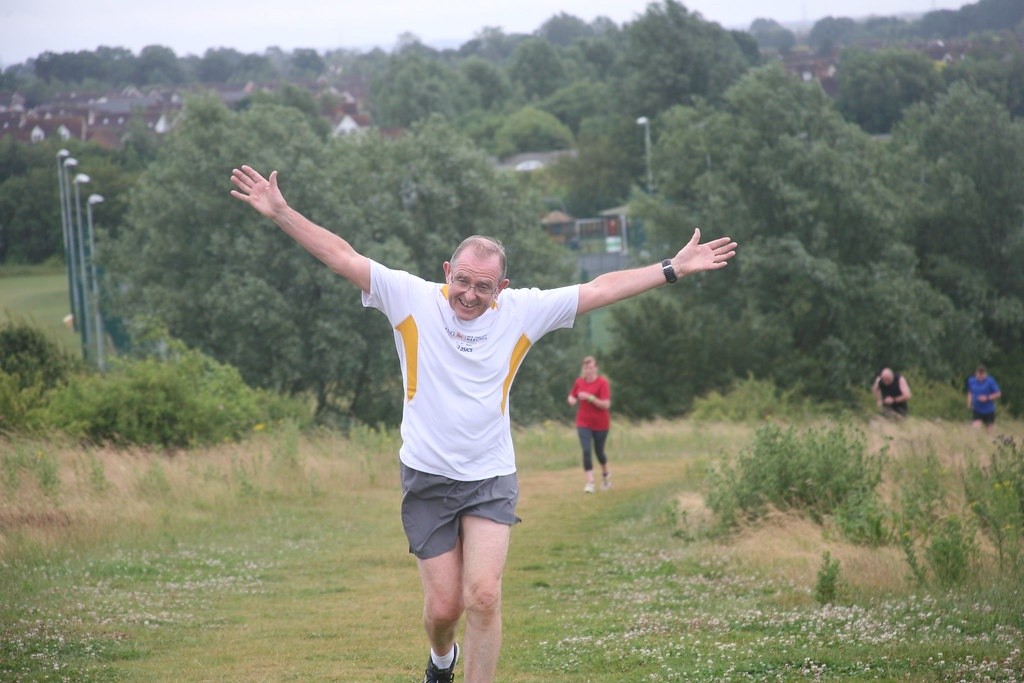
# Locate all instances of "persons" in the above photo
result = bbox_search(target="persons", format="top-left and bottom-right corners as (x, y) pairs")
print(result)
(567, 355), (612, 492)
(229, 164), (736, 683)
(966, 363), (1001, 433)
(873, 367), (911, 423)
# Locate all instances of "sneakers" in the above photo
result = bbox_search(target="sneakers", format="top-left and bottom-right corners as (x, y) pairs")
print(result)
(423, 641), (460, 683)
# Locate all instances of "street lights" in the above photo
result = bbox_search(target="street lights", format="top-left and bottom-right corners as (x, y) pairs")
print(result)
(55, 148), (78, 339)
(62, 157), (90, 366)
(637, 114), (653, 196)
(85, 193), (106, 375)
(72, 173), (92, 363)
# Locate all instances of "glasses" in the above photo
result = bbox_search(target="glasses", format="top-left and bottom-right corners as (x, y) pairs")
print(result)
(450, 265), (501, 299)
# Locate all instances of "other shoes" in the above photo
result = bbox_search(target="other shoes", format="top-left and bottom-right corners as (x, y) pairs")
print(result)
(601, 478), (612, 491)
(585, 480), (596, 493)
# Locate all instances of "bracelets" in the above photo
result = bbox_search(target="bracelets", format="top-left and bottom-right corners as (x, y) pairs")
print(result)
(662, 258), (677, 284)
(588, 394), (595, 403)
(987, 396), (990, 401)
